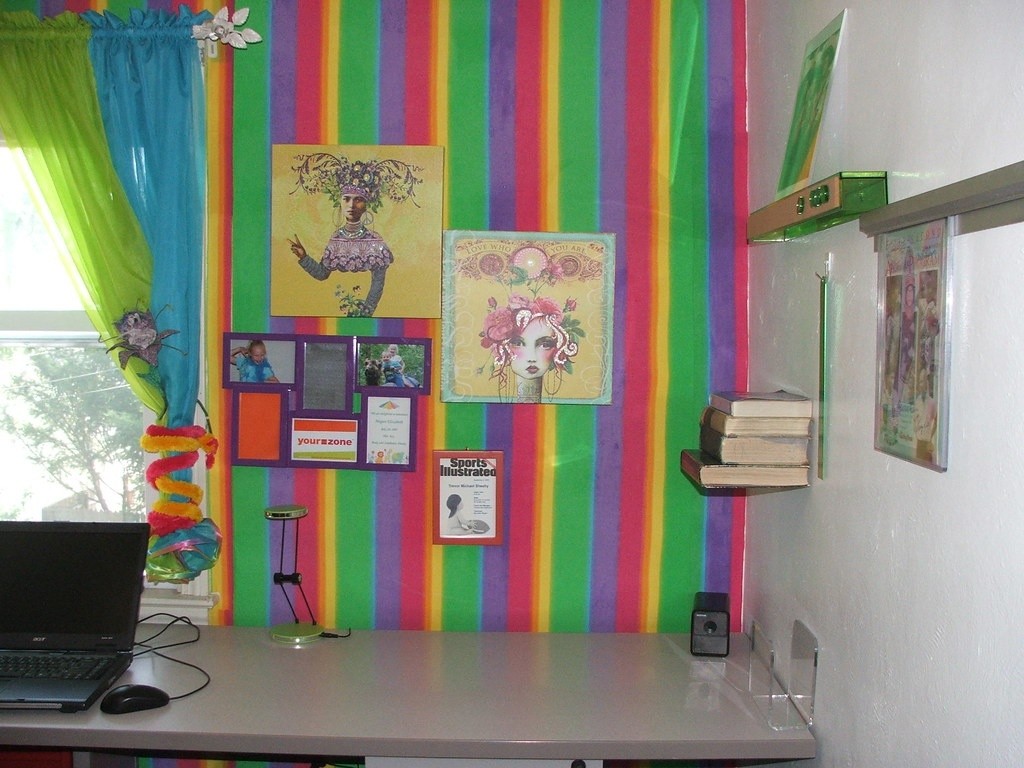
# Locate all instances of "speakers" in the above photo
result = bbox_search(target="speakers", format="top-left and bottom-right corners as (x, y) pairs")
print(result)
(690, 592), (731, 657)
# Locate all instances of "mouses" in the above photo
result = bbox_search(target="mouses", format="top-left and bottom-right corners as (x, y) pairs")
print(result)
(100, 684), (169, 714)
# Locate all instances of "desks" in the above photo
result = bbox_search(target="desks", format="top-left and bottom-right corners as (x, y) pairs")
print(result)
(0, 622), (818, 768)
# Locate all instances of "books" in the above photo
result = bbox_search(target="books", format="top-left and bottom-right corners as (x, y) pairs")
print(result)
(681, 390), (814, 491)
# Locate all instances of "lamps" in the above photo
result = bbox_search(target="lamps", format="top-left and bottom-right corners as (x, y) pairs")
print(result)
(264, 506), (353, 644)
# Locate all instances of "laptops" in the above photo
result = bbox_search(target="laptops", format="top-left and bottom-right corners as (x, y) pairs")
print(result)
(0, 521), (150, 713)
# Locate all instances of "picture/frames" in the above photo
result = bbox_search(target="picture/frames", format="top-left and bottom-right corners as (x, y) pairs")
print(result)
(431, 450), (505, 546)
(873, 217), (954, 474)
(222, 331), (432, 473)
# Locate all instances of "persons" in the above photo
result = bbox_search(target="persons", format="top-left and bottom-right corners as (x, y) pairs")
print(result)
(230, 340), (279, 383)
(381, 344), (412, 387)
(882, 368), (900, 447)
(446, 494), (478, 536)
(912, 369), (937, 463)
(893, 285), (914, 404)
